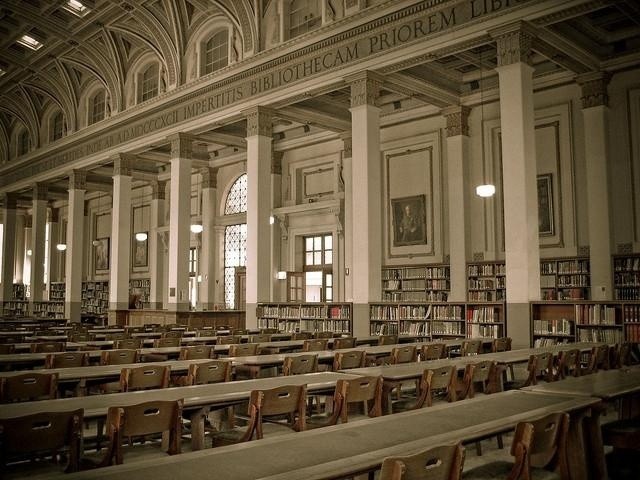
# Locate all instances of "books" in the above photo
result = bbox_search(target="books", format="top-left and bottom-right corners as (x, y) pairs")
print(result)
(4, 280), (149, 319)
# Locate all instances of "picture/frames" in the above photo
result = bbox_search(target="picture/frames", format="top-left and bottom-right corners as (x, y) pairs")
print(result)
(538, 171), (557, 238)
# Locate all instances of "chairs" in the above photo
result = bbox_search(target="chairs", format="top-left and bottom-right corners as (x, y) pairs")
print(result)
(0, 321), (639, 480)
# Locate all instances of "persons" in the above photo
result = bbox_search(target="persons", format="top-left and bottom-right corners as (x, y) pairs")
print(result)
(399, 205), (417, 242)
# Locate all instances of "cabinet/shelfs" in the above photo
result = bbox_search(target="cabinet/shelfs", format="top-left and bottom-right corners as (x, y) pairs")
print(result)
(2, 278), (153, 320)
(369, 261), (506, 338)
(529, 255), (640, 347)
(256, 301), (353, 339)
